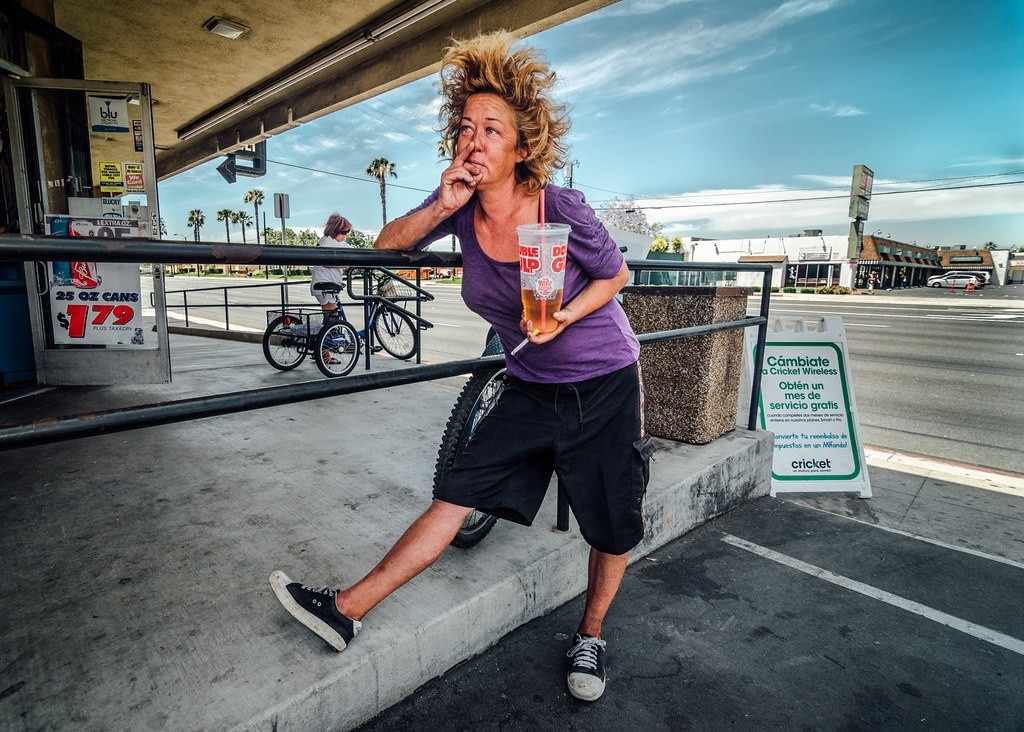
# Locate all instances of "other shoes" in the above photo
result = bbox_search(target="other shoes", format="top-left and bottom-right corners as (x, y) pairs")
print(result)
(321, 351), (341, 364)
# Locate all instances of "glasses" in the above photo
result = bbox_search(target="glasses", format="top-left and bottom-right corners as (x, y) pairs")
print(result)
(337, 229), (350, 235)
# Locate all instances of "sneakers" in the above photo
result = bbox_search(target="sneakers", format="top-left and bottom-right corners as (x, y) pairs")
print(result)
(268, 571), (362, 653)
(567, 633), (607, 702)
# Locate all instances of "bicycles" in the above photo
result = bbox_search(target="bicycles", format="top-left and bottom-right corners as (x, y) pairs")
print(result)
(432, 245), (628, 548)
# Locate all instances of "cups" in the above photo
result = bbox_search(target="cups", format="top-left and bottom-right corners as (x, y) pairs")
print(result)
(516, 223), (572, 333)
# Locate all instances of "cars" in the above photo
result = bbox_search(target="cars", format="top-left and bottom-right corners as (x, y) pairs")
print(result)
(434, 268), (450, 279)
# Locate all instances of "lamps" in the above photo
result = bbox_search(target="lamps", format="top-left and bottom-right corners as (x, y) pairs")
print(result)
(175, 0), (458, 141)
(871, 229), (931, 249)
(201, 16), (250, 40)
(125, 94), (157, 105)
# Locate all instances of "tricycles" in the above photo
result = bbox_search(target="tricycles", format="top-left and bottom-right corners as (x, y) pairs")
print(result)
(262, 268), (419, 378)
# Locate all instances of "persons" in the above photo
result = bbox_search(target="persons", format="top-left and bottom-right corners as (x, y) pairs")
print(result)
(267, 27), (656, 703)
(310, 212), (355, 364)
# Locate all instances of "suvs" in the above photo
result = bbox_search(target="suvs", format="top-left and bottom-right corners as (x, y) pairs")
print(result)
(927, 271), (992, 286)
(927, 275), (980, 289)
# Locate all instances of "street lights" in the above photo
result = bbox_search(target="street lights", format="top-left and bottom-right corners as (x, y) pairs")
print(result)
(173, 232), (194, 278)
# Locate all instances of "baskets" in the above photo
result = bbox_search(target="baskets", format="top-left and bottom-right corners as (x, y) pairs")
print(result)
(368, 277), (412, 298)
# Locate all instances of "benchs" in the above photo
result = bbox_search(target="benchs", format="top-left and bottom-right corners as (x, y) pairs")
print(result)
(797, 278), (839, 287)
